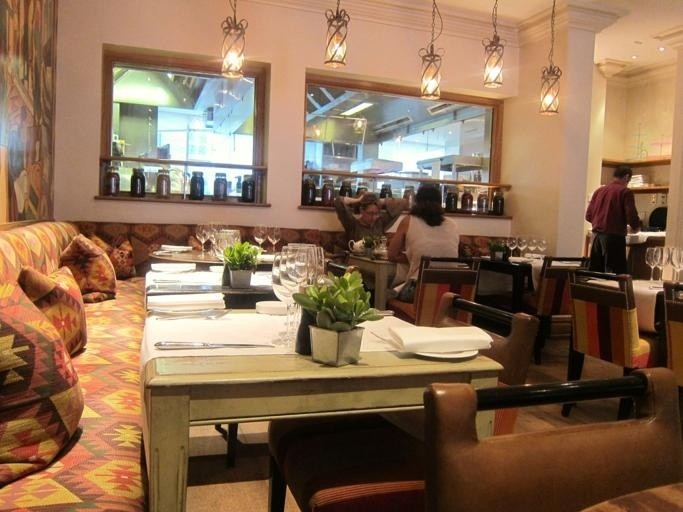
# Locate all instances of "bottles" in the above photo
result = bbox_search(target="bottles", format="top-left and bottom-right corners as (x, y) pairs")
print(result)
(430, 158), (440, 179)
(476, 170), (481, 181)
(451, 163), (456, 180)
(486, 238), (506, 260)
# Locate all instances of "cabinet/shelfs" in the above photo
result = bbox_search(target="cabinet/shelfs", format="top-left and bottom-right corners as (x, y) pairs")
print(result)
(600, 155), (671, 195)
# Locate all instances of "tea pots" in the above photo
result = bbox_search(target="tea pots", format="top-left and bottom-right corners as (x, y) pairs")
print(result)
(371, 235), (390, 260)
(347, 238), (367, 256)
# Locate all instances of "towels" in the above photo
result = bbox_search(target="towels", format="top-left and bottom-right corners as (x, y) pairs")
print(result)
(161, 245), (192, 252)
(148, 291), (226, 314)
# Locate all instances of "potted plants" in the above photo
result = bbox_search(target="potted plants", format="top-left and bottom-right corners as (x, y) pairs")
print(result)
(220, 242), (259, 288)
(291, 271), (385, 366)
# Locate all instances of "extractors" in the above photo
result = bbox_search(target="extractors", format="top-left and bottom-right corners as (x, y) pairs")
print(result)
(304, 84), (343, 116)
(111, 68), (194, 108)
(416, 122), (485, 170)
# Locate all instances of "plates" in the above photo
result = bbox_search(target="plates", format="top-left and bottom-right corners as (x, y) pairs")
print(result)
(509, 256), (530, 262)
(254, 301), (287, 315)
(210, 265), (226, 274)
(627, 174), (649, 187)
(256, 254), (274, 262)
(161, 244), (192, 253)
(409, 350), (479, 359)
(481, 255), (494, 260)
(152, 306), (217, 317)
(648, 206), (666, 231)
(150, 263), (194, 275)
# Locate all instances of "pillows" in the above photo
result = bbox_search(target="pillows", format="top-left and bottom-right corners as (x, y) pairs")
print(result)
(110, 236), (136, 279)
(17, 264), (87, 356)
(0, 281), (85, 486)
(86, 232), (113, 258)
(60, 232), (117, 303)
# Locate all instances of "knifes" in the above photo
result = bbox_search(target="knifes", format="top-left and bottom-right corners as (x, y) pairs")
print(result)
(153, 343), (278, 352)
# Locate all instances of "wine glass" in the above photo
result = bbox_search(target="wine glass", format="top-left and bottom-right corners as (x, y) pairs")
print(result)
(644, 246), (682, 288)
(268, 224), (280, 252)
(253, 222), (268, 249)
(536, 238), (547, 258)
(272, 242), (325, 349)
(527, 237), (537, 257)
(506, 233), (516, 257)
(516, 235), (528, 257)
(195, 222), (209, 257)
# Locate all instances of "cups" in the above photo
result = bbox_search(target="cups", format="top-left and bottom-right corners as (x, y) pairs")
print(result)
(207, 222), (222, 242)
(213, 228), (240, 262)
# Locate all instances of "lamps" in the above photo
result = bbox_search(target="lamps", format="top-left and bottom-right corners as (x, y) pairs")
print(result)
(220, 0), (248, 78)
(417, 0), (445, 101)
(538, 0), (562, 116)
(324, 0), (350, 69)
(482, 0), (507, 90)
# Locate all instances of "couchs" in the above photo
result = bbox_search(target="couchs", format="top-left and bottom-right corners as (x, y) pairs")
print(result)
(145, 223), (489, 257)
(0, 220), (147, 512)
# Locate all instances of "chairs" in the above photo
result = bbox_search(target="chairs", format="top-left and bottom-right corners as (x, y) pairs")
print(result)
(268, 291), (539, 512)
(387, 236), (683, 512)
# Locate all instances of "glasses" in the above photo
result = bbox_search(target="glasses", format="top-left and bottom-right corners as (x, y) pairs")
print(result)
(363, 209), (379, 216)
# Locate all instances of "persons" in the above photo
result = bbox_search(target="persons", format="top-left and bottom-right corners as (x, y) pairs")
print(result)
(585, 165), (643, 274)
(386, 184), (460, 302)
(333, 191), (409, 254)
